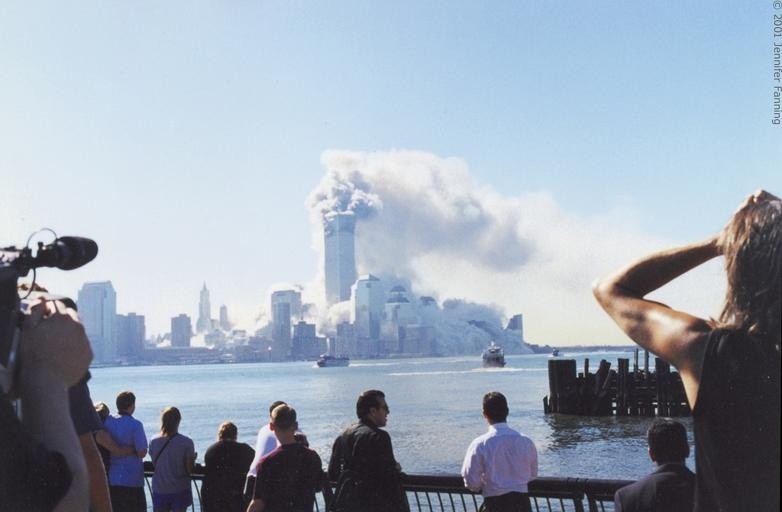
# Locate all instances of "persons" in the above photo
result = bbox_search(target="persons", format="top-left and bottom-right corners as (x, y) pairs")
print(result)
(95, 401), (136, 474)
(610, 417), (696, 512)
(146, 403), (197, 512)
(14, 282), (115, 512)
(292, 429), (309, 448)
(0, 293), (94, 511)
(94, 390), (148, 511)
(328, 388), (406, 511)
(242, 400), (304, 512)
(200, 420), (256, 512)
(244, 406), (327, 512)
(589, 184), (782, 511)
(460, 391), (539, 511)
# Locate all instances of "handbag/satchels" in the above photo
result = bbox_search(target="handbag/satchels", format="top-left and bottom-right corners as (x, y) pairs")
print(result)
(332, 429), (386, 511)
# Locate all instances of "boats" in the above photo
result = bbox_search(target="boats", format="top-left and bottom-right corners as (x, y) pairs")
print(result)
(483, 339), (507, 369)
(548, 348), (564, 357)
(317, 352), (351, 369)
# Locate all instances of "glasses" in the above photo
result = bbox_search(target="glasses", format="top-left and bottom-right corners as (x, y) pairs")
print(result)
(378, 406), (388, 412)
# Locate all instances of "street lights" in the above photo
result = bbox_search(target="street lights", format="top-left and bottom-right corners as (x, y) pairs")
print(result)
(268, 346), (272, 361)
